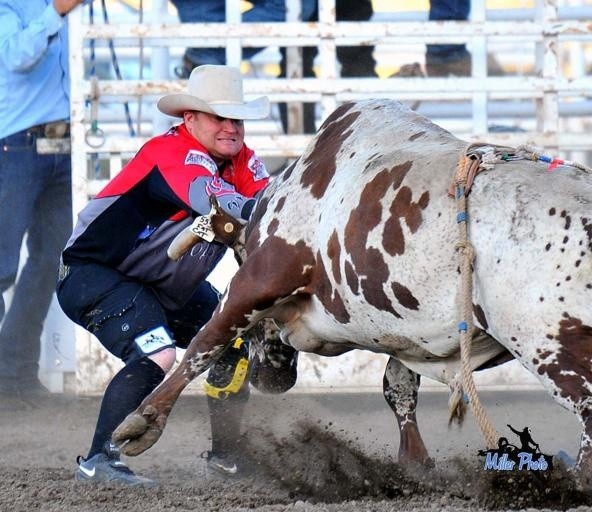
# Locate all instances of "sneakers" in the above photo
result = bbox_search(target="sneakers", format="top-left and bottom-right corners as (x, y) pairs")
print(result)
(73, 440), (155, 487)
(201, 449), (254, 483)
(0, 385), (73, 413)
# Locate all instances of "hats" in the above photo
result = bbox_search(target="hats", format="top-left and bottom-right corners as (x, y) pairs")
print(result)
(157, 65), (272, 120)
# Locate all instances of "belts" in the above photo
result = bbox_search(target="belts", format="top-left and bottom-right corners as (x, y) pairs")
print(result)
(37, 121), (71, 139)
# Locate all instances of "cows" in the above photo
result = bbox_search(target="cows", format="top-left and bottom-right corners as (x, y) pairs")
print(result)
(109, 96), (592, 512)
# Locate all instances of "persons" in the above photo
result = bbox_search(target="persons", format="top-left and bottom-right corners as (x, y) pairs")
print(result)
(170, 1), (317, 80)
(424, 0), (507, 79)
(54, 62), (271, 493)
(276, 0), (385, 134)
(0, 0), (85, 413)
